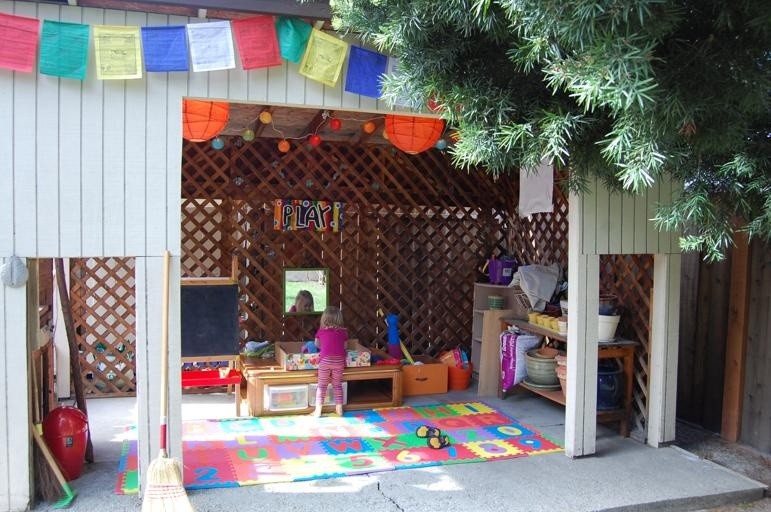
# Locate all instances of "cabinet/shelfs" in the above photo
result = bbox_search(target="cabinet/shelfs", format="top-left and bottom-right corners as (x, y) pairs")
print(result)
(470, 281), (517, 381)
(498, 319), (633, 438)
(240, 354), (403, 416)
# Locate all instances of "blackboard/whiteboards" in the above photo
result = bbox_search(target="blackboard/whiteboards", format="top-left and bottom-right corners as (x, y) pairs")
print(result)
(181, 280), (240, 363)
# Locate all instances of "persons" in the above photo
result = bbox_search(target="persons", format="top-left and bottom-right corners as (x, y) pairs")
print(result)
(290, 290), (313, 312)
(311, 306), (349, 417)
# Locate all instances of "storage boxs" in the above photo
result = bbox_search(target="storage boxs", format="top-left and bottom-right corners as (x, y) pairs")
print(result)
(401, 355), (449, 396)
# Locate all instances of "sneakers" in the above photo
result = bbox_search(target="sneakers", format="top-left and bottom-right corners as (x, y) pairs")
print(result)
(416, 425), (441, 438)
(427, 435), (450, 449)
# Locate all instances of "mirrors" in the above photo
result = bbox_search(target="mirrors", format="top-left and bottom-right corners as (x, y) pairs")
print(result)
(283, 267), (330, 317)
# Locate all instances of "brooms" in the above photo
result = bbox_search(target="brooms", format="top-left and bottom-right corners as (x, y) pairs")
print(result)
(31, 347), (80, 509)
(141, 250), (198, 512)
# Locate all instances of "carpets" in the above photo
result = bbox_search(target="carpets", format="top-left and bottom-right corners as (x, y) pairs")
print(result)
(115, 398), (567, 495)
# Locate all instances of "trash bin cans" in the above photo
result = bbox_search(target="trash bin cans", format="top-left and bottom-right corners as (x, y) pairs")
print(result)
(44, 405), (88, 480)
